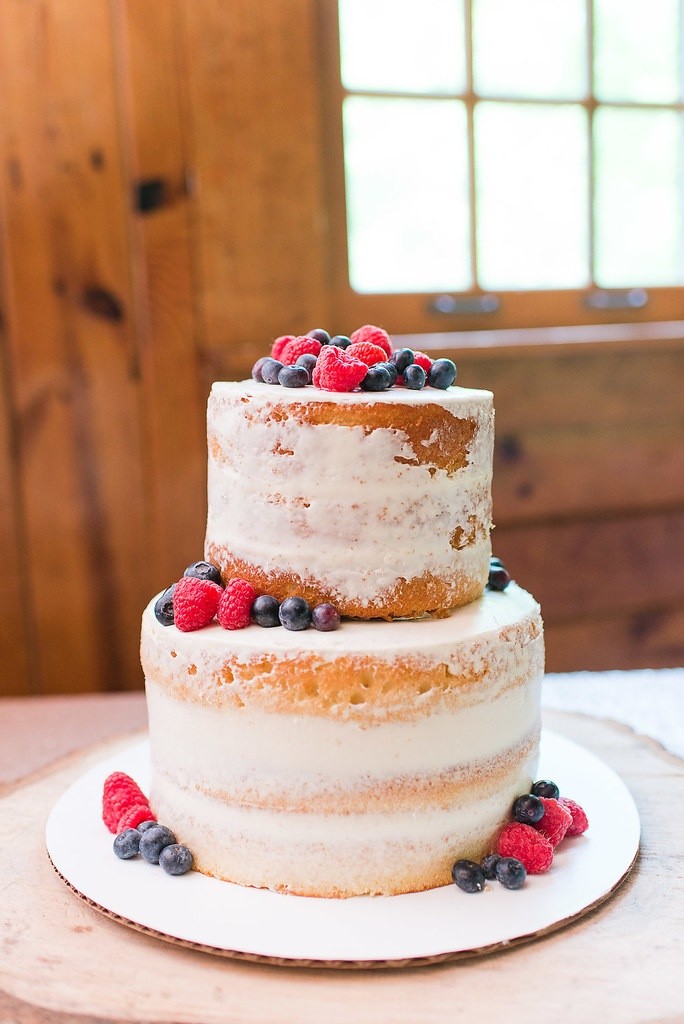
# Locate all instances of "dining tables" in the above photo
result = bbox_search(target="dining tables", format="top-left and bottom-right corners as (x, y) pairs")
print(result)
(0, 664), (684, 1024)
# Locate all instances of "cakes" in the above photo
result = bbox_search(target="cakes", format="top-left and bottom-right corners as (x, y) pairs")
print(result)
(99, 326), (590, 894)
